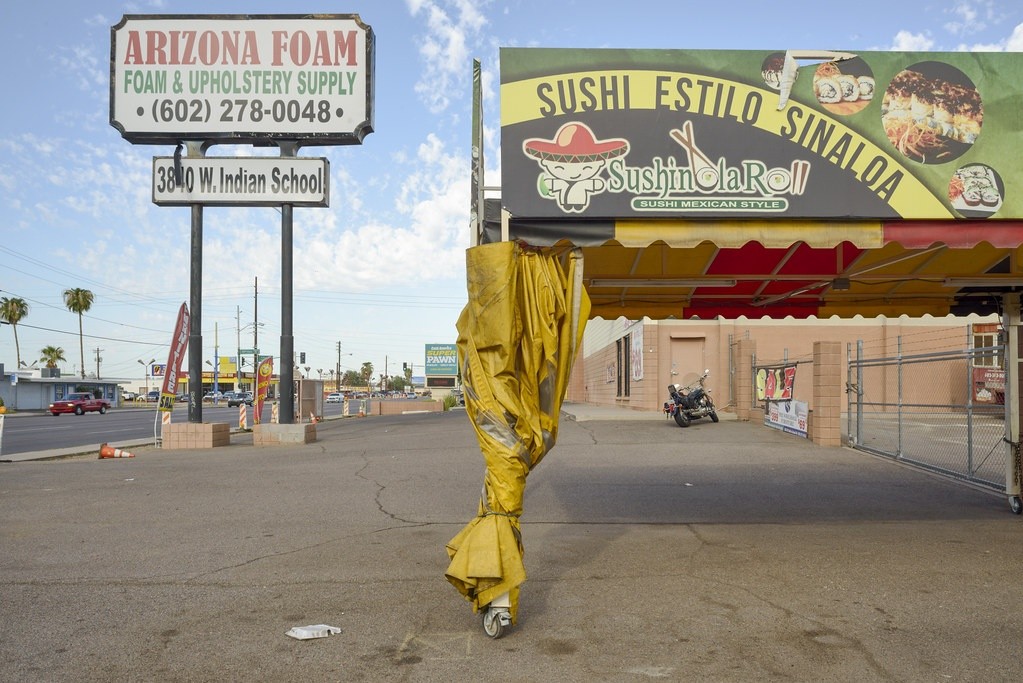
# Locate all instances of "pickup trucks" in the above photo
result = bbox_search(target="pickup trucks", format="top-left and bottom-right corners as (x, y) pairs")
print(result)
(49, 391), (112, 416)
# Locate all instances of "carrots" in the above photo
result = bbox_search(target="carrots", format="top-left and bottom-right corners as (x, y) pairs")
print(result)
(949, 174), (966, 201)
(882, 112), (948, 161)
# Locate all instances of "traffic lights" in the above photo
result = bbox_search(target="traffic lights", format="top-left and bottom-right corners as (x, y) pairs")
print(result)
(300, 352), (306, 365)
(242, 357), (246, 366)
(402, 362), (408, 371)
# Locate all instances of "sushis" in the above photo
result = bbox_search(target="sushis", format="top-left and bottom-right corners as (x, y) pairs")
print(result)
(883, 69), (983, 145)
(812, 73), (875, 104)
(958, 168), (1000, 207)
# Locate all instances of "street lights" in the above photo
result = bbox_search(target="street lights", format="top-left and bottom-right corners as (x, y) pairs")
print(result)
(304, 366), (311, 379)
(205, 359), (219, 405)
(338, 353), (353, 392)
(329, 369), (334, 384)
(317, 368), (323, 380)
(379, 374), (388, 390)
(237, 322), (265, 389)
(384, 355), (397, 376)
(137, 358), (156, 403)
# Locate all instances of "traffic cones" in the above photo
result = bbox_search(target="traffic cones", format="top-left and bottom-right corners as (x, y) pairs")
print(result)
(98, 442), (137, 458)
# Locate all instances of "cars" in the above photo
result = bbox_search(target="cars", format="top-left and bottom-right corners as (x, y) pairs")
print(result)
(228, 393), (254, 407)
(346, 391), (418, 399)
(119, 390), (255, 402)
(327, 393), (345, 403)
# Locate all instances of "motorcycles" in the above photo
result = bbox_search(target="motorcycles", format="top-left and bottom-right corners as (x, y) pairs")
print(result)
(663, 369), (719, 427)
